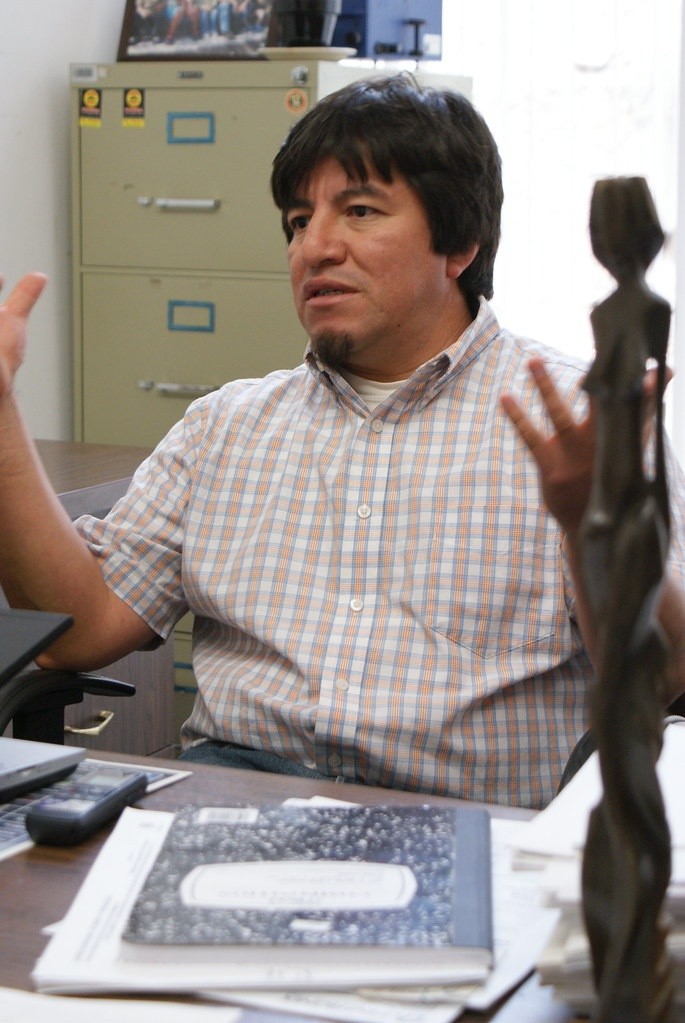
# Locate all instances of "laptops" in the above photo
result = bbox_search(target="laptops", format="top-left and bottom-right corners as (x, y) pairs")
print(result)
(0, 607), (88, 802)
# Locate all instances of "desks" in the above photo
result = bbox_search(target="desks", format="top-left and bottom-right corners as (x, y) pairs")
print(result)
(0, 736), (544, 1023)
(0, 437), (177, 760)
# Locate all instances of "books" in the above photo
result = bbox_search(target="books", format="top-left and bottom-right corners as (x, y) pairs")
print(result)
(510, 720), (685, 1023)
(116, 807), (493, 972)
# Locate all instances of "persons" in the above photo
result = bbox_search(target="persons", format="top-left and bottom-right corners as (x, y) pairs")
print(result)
(0, 73), (685, 811)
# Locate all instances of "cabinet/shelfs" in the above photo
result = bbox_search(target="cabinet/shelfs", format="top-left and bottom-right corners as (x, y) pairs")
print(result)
(66, 59), (474, 693)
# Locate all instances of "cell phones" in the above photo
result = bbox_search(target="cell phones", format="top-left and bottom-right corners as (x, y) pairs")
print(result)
(23, 766), (146, 849)
